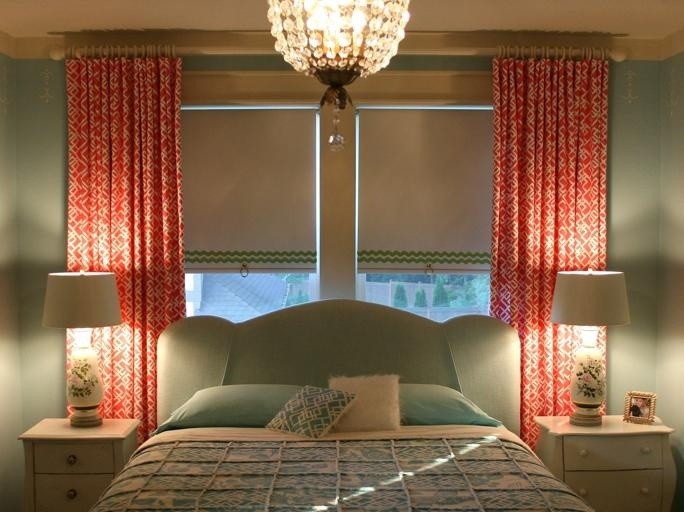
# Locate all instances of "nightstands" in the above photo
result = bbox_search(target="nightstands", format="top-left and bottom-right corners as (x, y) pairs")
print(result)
(533, 415), (678, 512)
(18, 418), (142, 512)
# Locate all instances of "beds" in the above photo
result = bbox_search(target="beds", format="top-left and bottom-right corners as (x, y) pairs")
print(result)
(89, 298), (593, 512)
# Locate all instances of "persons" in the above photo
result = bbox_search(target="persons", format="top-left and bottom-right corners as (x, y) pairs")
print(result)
(630, 397), (641, 416)
(638, 398), (649, 418)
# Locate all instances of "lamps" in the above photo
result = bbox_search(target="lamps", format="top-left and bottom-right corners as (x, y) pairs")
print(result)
(43, 271), (121, 427)
(266, 0), (411, 149)
(551, 267), (631, 426)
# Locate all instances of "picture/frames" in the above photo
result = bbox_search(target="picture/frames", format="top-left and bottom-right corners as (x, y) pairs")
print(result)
(623, 391), (656, 425)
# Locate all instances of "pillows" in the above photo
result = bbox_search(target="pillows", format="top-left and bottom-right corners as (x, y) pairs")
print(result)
(398, 383), (501, 427)
(267, 385), (358, 439)
(328, 375), (400, 432)
(150, 384), (306, 435)
